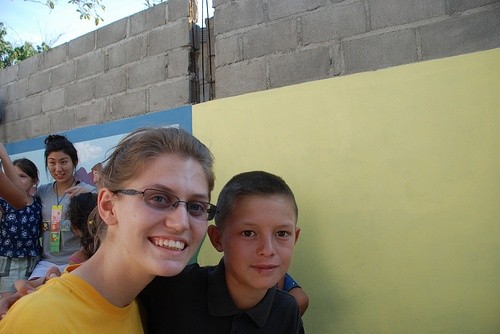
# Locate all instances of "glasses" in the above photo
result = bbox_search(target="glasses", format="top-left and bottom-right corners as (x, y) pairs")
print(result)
(111, 188), (219, 221)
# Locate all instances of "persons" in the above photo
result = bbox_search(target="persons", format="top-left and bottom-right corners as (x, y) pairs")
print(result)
(28, 134), (97, 281)
(67, 192), (100, 266)
(0, 127), (219, 334)
(0, 158), (43, 299)
(0, 171), (306, 334)
(0, 143), (27, 210)
